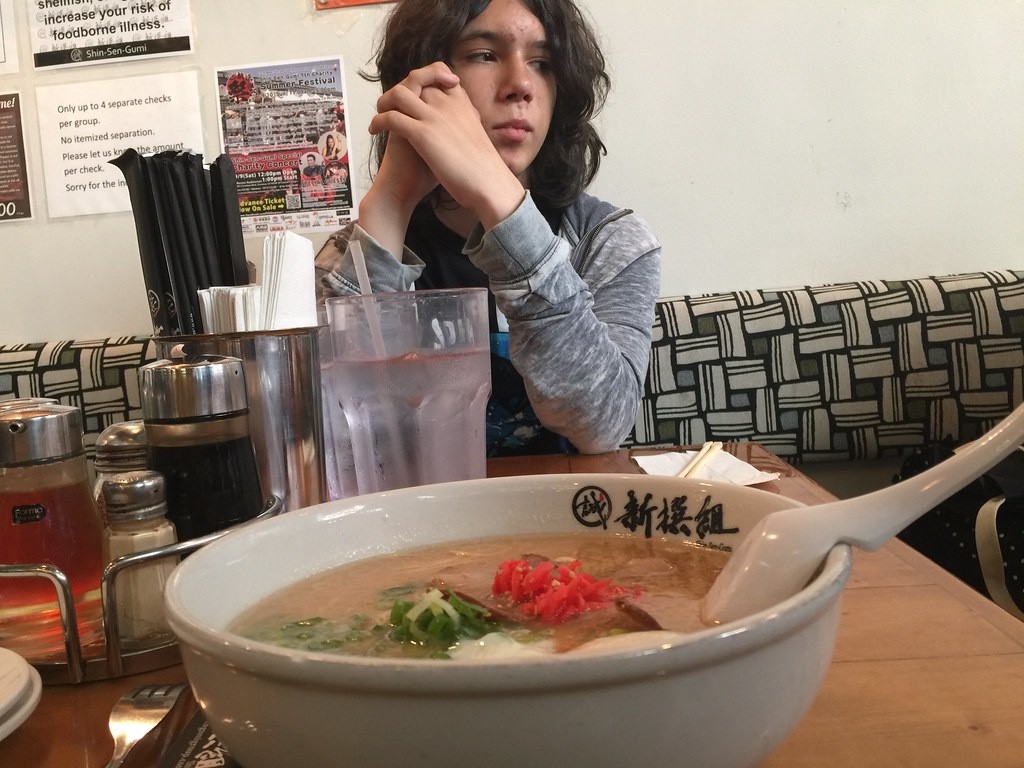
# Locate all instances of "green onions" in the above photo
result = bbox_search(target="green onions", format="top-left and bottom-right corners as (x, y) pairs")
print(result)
(248, 590), (506, 662)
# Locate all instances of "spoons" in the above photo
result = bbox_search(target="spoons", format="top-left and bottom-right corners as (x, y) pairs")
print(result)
(702, 403), (1024, 625)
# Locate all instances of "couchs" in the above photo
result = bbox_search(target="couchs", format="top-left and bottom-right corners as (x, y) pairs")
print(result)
(2, 267), (1024, 544)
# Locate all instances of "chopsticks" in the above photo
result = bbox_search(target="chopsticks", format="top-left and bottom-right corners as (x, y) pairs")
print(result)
(676, 441), (723, 478)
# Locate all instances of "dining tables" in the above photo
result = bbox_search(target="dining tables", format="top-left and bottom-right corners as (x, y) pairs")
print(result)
(0, 443), (1024, 767)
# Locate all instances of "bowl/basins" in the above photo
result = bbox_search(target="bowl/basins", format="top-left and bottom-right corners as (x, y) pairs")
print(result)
(160, 473), (853, 768)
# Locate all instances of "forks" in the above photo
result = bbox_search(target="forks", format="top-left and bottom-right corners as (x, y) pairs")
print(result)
(105, 683), (188, 768)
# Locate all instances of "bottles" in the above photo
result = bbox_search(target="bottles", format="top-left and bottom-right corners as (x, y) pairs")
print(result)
(0, 398), (60, 406)
(94, 419), (152, 530)
(0, 404), (106, 659)
(138, 354), (261, 564)
(100, 470), (182, 651)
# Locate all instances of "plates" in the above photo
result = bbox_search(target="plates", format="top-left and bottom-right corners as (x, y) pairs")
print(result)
(0, 665), (43, 741)
(0, 647), (31, 717)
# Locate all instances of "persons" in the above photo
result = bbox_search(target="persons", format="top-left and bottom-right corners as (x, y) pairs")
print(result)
(304, 133), (347, 184)
(288, 0), (661, 458)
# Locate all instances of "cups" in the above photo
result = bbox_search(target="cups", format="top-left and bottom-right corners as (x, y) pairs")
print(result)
(326, 287), (491, 501)
(318, 305), (379, 372)
(141, 323), (327, 517)
